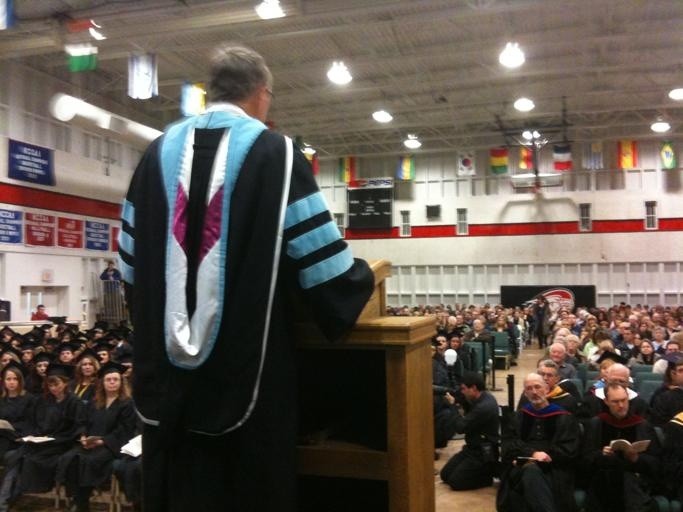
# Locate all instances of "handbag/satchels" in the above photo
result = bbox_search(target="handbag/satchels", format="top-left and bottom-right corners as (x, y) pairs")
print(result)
(470, 439), (499, 472)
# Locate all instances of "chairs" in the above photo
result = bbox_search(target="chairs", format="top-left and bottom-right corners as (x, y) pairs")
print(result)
(564, 364), (666, 406)
(465, 331), (512, 390)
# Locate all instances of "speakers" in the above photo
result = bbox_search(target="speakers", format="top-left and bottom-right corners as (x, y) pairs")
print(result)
(427, 206), (438, 216)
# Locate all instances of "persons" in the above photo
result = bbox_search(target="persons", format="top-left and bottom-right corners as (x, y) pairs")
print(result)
(0, 304), (144, 512)
(100, 261), (132, 325)
(117, 45), (395, 510)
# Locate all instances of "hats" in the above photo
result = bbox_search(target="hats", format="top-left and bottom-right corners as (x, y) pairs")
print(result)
(596, 351), (628, 364)
(666, 351), (683, 366)
(1, 321), (131, 379)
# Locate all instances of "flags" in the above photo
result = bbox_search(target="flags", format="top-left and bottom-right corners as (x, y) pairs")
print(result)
(300, 151), (319, 175)
(62, 34), (208, 118)
